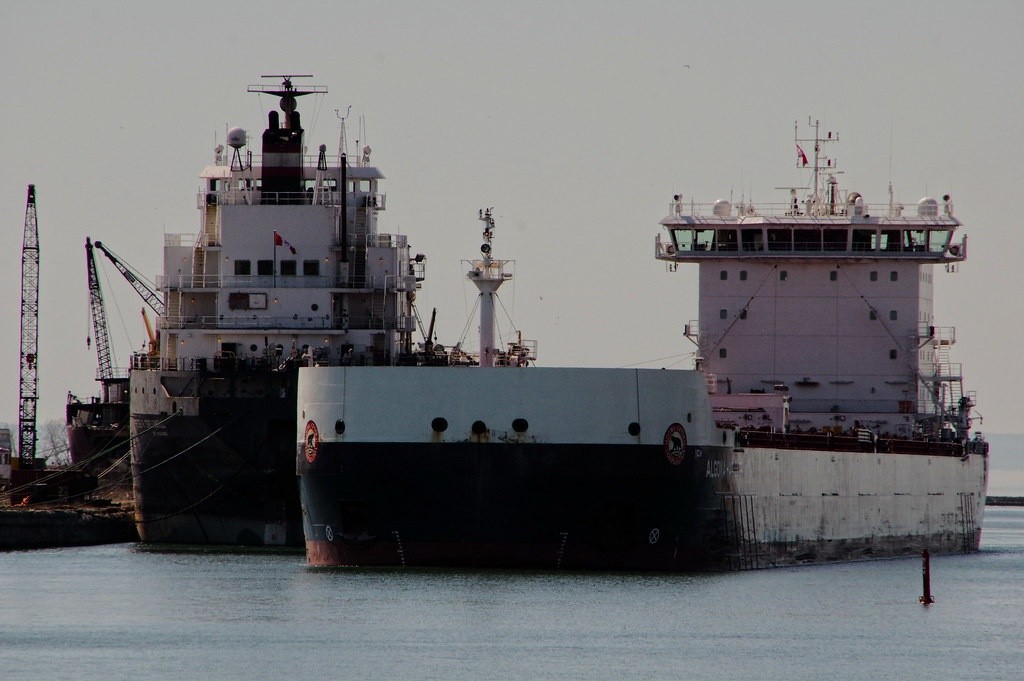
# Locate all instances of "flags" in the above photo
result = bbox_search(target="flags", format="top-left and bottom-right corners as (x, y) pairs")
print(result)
(796, 145), (809, 166)
(857, 428), (876, 446)
(275, 233), (296, 254)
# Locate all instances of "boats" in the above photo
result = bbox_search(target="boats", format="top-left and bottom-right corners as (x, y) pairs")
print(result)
(128, 74), (538, 546)
(295, 115), (991, 577)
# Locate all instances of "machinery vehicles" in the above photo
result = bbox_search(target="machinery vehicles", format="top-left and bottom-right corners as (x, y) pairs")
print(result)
(85, 237), (113, 397)
